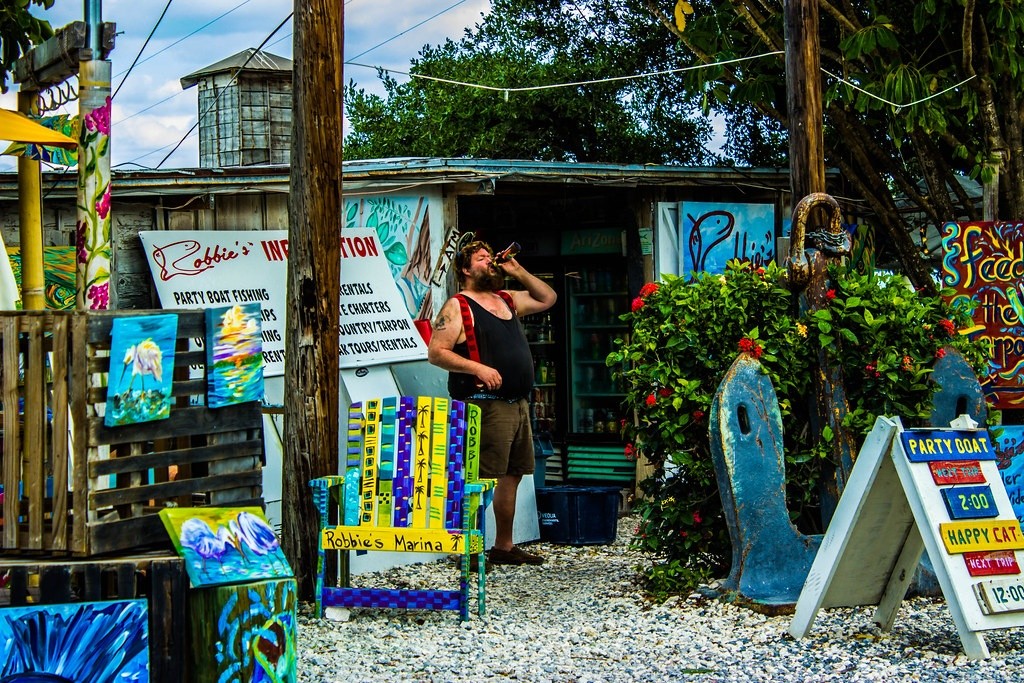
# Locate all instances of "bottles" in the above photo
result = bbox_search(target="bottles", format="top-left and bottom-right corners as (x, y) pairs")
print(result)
(579, 297), (625, 325)
(585, 365), (626, 393)
(573, 269), (628, 292)
(520, 312), (553, 341)
(532, 354), (557, 384)
(585, 331), (622, 360)
(577, 409), (621, 433)
(491, 242), (521, 269)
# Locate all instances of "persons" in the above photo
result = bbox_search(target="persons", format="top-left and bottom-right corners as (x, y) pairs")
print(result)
(425, 239), (557, 566)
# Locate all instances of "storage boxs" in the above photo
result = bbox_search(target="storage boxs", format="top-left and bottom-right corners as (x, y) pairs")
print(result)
(536, 484), (622, 546)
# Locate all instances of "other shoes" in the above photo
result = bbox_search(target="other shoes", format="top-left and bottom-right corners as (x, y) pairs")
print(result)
(456, 556), (493, 573)
(488, 546), (544, 566)
